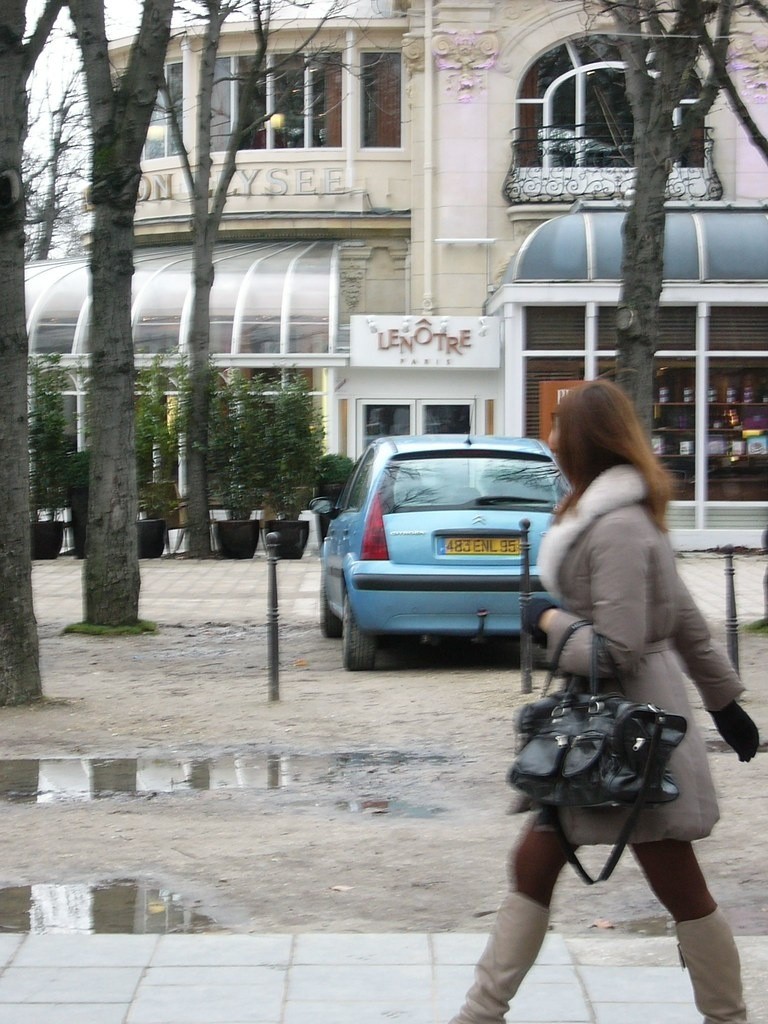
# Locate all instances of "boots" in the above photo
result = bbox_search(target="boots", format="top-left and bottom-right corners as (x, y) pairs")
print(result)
(448, 890), (549, 1024)
(675, 903), (748, 1024)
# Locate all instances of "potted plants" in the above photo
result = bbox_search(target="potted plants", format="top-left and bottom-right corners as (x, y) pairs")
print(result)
(265, 366), (325, 559)
(316, 453), (355, 546)
(25, 353), (65, 559)
(66, 449), (91, 558)
(136, 355), (190, 559)
(208, 372), (265, 560)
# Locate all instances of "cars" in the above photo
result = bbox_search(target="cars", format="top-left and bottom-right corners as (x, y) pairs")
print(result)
(310, 435), (572, 672)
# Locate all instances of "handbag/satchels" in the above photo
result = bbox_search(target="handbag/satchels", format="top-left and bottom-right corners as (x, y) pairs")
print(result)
(508, 620), (688, 885)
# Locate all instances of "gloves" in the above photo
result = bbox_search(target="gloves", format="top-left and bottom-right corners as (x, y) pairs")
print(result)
(705, 699), (760, 762)
(519, 592), (562, 641)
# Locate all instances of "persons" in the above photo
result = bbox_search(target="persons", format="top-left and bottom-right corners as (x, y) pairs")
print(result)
(447, 379), (759, 1024)
